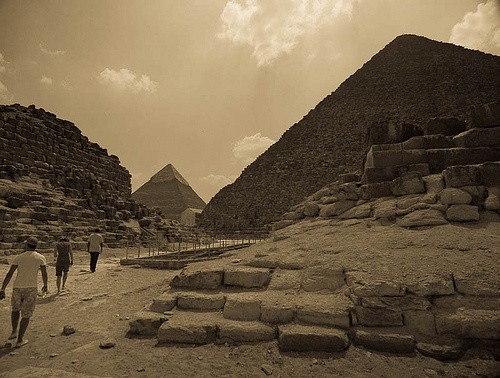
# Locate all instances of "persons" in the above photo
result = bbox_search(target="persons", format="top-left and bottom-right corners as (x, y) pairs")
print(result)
(53, 236), (73, 293)
(87, 228), (106, 273)
(0, 236), (48, 348)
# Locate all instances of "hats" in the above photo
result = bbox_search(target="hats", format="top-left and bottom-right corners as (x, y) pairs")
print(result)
(24, 237), (38, 245)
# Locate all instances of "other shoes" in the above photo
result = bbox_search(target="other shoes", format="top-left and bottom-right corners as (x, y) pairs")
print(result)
(15, 338), (28, 347)
(8, 333), (17, 340)
